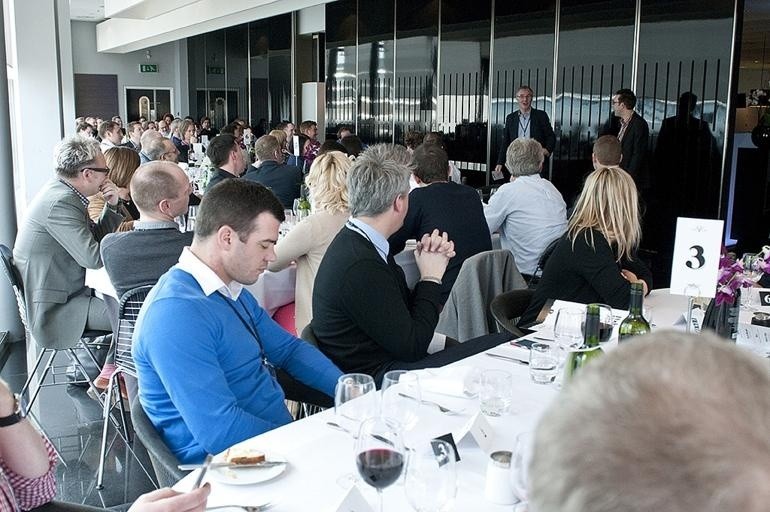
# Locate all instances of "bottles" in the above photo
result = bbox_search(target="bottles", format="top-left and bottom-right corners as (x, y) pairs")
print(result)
(569, 283), (650, 377)
(297, 184), (313, 220)
(188, 143), (196, 167)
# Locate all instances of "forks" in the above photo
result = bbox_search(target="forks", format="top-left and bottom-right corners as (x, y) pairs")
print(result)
(203, 503), (271, 512)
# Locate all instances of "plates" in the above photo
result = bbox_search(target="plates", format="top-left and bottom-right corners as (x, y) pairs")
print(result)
(206, 452), (289, 485)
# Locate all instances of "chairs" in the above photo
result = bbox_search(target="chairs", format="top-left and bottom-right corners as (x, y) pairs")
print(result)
(1, 244), (122, 430)
(528, 238), (559, 288)
(96, 285), (157, 489)
(296, 323), (328, 420)
(130, 365), (334, 488)
(436, 250), (514, 341)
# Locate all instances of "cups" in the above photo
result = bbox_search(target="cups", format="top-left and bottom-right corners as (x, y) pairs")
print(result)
(464, 342), (559, 419)
(555, 303), (614, 349)
(177, 203), (201, 234)
(335, 370), (423, 436)
(177, 161), (214, 193)
(281, 197), (299, 231)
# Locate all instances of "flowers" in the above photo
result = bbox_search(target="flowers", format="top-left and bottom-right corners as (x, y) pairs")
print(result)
(713, 240), (770, 305)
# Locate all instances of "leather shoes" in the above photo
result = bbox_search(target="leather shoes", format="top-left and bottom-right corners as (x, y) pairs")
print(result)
(86, 375), (130, 411)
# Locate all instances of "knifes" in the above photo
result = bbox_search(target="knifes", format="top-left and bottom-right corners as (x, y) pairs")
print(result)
(177, 461), (286, 468)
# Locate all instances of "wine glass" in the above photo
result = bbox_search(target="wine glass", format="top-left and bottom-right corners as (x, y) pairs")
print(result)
(356, 419), (406, 511)
(739, 251), (766, 315)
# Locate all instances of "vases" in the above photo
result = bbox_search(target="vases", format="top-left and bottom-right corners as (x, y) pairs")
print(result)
(700, 288), (740, 346)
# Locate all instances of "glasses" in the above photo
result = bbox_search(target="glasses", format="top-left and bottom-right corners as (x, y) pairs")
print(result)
(80, 166), (110, 176)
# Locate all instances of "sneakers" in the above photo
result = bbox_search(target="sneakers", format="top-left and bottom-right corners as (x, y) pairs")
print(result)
(66, 372), (93, 388)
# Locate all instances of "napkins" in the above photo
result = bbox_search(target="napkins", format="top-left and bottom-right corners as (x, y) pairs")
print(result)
(407, 367), (478, 399)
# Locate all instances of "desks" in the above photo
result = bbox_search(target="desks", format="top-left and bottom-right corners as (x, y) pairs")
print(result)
(85, 223), (295, 320)
